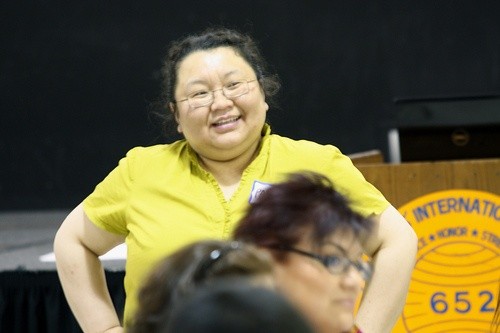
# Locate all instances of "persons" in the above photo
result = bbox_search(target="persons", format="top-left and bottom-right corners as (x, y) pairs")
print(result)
(121, 168), (363, 333)
(53, 29), (418, 333)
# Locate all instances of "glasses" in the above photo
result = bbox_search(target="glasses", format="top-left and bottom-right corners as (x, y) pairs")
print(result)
(173, 78), (258, 109)
(266, 241), (370, 285)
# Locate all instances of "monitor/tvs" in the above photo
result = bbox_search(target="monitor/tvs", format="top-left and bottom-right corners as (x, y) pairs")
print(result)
(394, 94), (500, 162)
